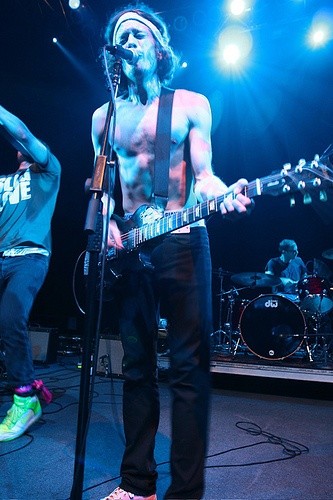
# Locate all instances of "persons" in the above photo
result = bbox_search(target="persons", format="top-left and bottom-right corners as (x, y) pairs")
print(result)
(265, 239), (309, 305)
(1, 106), (61, 442)
(91, 5), (255, 500)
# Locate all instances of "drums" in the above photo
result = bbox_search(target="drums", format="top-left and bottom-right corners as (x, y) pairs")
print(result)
(238, 293), (307, 362)
(296, 275), (333, 314)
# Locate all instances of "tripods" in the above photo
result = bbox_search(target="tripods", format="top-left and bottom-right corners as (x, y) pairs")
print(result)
(211, 276), (247, 351)
(302, 295), (332, 359)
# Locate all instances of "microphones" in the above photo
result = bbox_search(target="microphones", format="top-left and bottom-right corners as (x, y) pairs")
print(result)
(105, 44), (139, 65)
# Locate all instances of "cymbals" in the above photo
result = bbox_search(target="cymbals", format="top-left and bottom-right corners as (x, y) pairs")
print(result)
(320, 248), (332, 259)
(211, 268), (234, 278)
(230, 270), (282, 288)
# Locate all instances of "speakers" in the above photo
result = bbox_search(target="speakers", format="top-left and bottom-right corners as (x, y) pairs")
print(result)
(28, 327), (59, 364)
(157, 329), (172, 356)
(98, 335), (127, 378)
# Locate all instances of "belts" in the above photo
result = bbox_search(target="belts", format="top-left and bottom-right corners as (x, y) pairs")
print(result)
(189, 219), (207, 228)
(2, 247), (50, 258)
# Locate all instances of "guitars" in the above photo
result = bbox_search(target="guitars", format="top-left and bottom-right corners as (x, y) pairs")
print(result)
(82, 151), (329, 294)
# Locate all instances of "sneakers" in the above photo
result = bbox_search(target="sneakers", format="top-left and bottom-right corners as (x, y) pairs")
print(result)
(0, 394), (42, 441)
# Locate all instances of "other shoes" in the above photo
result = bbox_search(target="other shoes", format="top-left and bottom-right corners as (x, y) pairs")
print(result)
(99, 487), (158, 500)
